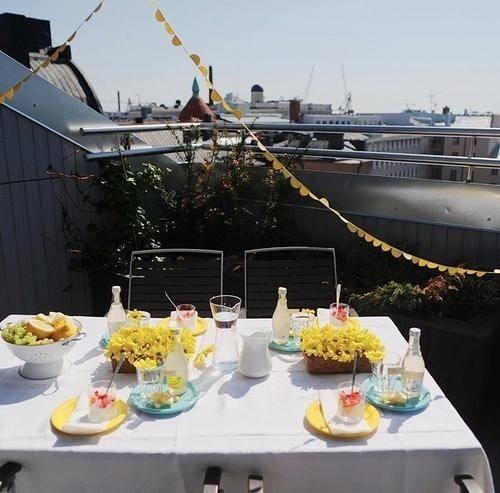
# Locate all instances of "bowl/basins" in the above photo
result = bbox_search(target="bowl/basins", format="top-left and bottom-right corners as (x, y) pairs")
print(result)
(1, 314), (86, 381)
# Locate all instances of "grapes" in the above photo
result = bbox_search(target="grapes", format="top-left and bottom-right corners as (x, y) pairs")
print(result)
(36, 337), (54, 344)
(2, 323), (37, 345)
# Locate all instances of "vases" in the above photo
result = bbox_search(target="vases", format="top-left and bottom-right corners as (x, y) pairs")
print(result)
(304, 347), (374, 376)
(109, 351), (187, 374)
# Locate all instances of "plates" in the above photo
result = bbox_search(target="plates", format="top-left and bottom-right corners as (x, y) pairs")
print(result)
(98, 323), (114, 348)
(267, 336), (303, 352)
(363, 373), (431, 411)
(303, 389), (383, 439)
(157, 315), (208, 335)
(265, 327), (319, 351)
(130, 377), (200, 414)
(49, 394), (128, 435)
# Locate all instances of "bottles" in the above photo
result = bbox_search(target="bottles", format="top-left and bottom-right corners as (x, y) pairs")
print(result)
(402, 328), (425, 399)
(272, 287), (290, 344)
(164, 328), (188, 395)
(106, 286), (127, 338)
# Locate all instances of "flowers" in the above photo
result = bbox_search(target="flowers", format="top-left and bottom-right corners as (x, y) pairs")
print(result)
(105, 324), (215, 371)
(298, 319), (385, 365)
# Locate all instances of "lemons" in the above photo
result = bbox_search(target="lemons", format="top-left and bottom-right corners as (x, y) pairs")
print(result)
(30, 319), (53, 338)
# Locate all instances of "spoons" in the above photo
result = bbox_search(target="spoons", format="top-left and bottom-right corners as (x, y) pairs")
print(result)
(335, 284), (340, 316)
(105, 353), (126, 395)
(349, 351), (360, 397)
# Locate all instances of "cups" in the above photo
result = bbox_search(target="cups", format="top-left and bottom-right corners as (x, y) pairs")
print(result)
(330, 302), (349, 328)
(135, 356), (164, 399)
(236, 328), (274, 378)
(372, 352), (401, 393)
(291, 313), (315, 344)
(175, 304), (197, 330)
(335, 380), (366, 422)
(86, 380), (117, 421)
(209, 294), (242, 372)
(128, 310), (150, 325)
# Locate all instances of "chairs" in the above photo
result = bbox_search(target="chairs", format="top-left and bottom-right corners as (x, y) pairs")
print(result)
(126, 250), (224, 320)
(0, 460), (224, 493)
(244, 474), (486, 493)
(244, 245), (339, 320)
